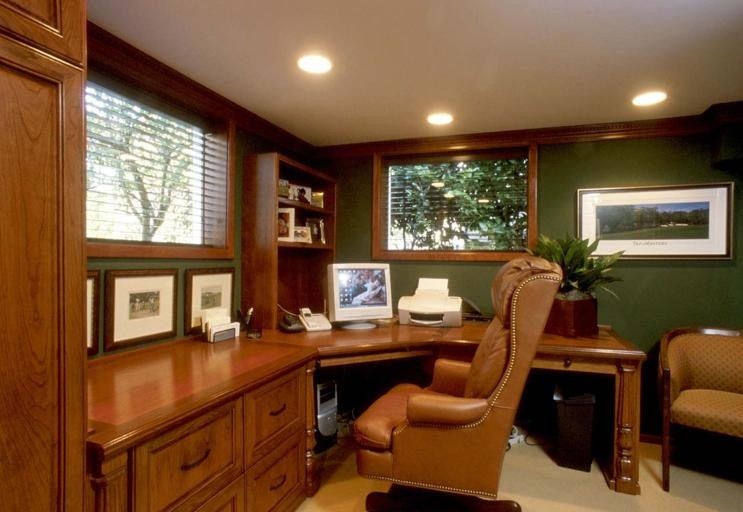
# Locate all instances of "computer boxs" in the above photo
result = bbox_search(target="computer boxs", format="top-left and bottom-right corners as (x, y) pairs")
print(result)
(315, 378), (338, 452)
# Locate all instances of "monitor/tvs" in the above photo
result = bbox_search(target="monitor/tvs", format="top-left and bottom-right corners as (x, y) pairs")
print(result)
(327, 262), (393, 329)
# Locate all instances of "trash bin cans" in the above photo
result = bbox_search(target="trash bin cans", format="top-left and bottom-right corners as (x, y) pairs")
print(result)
(553, 378), (595, 473)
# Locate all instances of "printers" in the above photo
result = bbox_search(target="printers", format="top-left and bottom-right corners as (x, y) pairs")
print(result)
(397, 278), (464, 328)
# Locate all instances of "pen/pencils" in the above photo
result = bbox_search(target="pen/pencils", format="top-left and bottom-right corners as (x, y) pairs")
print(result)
(236, 307), (254, 332)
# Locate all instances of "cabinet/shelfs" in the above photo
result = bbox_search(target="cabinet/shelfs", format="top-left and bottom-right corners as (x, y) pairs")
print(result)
(87, 335), (318, 512)
(240, 153), (338, 330)
(1, 0), (88, 512)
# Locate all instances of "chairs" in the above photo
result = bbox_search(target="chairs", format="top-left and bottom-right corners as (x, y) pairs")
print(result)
(354, 258), (563, 498)
(658, 325), (743, 493)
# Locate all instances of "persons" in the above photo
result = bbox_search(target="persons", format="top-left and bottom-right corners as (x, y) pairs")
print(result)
(278, 217), (289, 236)
(313, 223), (319, 239)
(351, 275), (383, 304)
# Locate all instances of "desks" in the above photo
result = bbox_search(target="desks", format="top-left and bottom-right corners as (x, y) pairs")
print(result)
(240, 324), (648, 498)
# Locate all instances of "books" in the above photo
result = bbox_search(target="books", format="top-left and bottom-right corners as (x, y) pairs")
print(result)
(211, 322), (240, 342)
(207, 317), (231, 341)
(202, 310), (230, 333)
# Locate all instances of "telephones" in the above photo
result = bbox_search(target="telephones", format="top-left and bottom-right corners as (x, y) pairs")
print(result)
(298, 307), (332, 331)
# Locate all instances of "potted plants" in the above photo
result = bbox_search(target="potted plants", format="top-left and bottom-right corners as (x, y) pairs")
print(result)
(534, 235), (625, 338)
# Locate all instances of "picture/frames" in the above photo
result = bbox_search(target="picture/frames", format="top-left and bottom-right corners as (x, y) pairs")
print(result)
(278, 208), (295, 242)
(306, 218), (325, 244)
(86, 269), (100, 357)
(103, 267), (180, 353)
(576, 181), (736, 263)
(289, 183), (311, 204)
(184, 267), (236, 337)
(295, 226), (312, 243)
